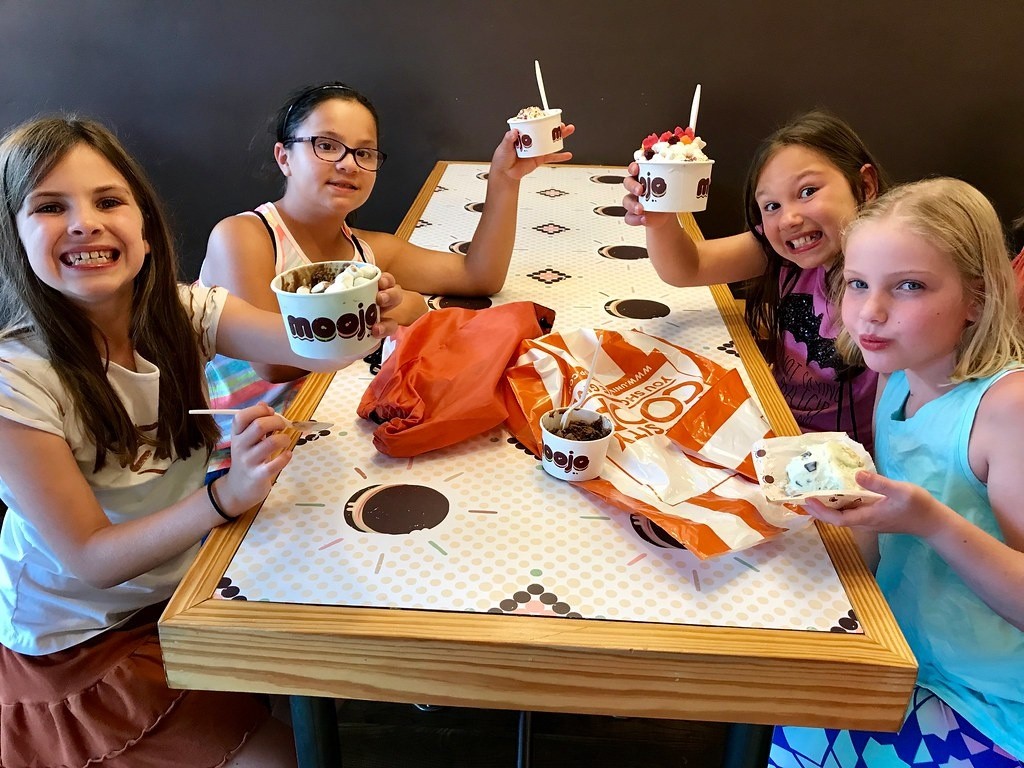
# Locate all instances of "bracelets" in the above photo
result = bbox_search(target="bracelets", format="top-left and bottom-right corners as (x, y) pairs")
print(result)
(207, 473), (241, 521)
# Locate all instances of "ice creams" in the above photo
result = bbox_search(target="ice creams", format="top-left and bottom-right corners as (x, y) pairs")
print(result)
(633, 127), (712, 162)
(545, 414), (612, 441)
(511, 105), (549, 120)
(776, 437), (875, 493)
(292, 263), (378, 294)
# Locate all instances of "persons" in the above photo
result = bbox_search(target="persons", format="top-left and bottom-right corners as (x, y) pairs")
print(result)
(198, 81), (574, 550)
(763, 176), (1023, 768)
(622, 111), (1024, 460)
(0, 115), (402, 768)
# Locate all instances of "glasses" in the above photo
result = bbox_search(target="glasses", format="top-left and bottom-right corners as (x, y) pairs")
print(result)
(285, 136), (387, 172)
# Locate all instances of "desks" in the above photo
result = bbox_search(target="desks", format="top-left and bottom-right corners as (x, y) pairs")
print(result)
(155, 160), (918, 768)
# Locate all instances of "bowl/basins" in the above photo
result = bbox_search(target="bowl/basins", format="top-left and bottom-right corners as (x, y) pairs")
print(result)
(635, 159), (715, 213)
(270, 261), (382, 360)
(539, 407), (614, 481)
(507, 109), (563, 158)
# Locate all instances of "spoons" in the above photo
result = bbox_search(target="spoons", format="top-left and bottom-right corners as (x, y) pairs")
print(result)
(560, 334), (604, 429)
(188, 409), (334, 433)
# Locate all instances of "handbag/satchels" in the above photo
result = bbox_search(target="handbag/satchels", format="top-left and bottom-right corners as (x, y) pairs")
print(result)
(357, 301), (556, 458)
(498, 327), (820, 562)
